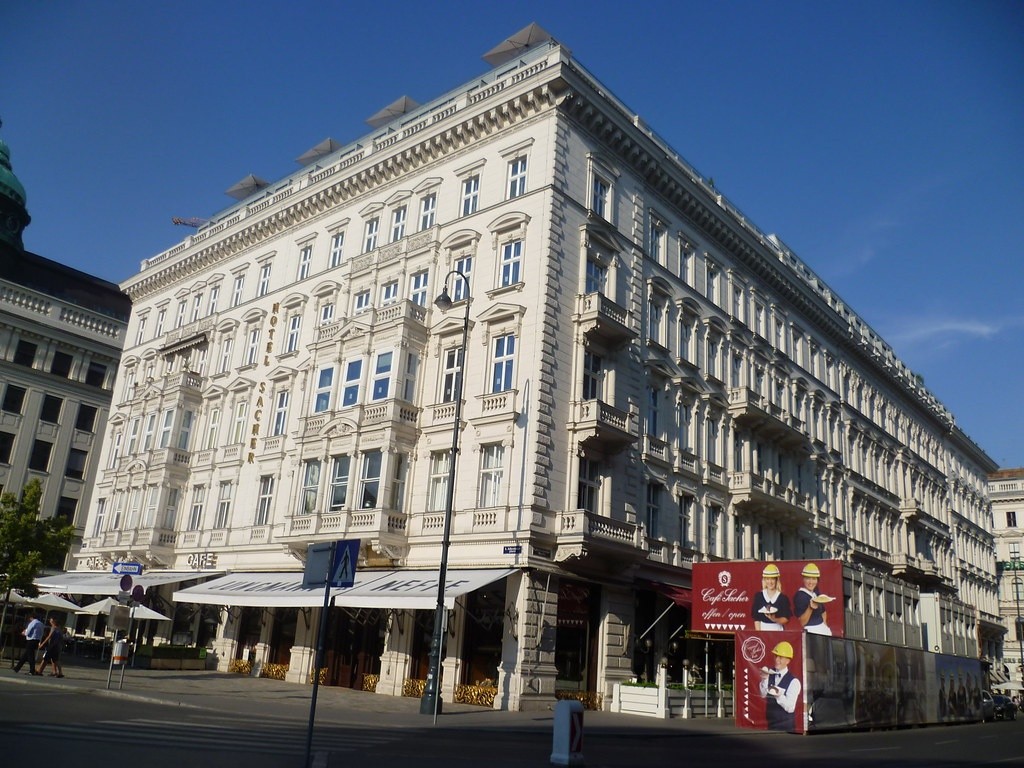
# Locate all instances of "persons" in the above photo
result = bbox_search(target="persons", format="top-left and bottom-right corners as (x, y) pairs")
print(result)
(12, 614), (44, 675)
(34, 616), (71, 679)
(753, 563), (833, 734)
(938, 663), (983, 716)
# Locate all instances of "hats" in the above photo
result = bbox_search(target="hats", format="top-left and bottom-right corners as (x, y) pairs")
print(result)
(958, 667), (963, 678)
(950, 672), (954, 680)
(940, 668), (945, 678)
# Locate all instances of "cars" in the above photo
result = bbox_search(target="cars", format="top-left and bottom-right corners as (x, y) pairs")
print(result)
(992, 695), (1018, 720)
(982, 690), (994, 720)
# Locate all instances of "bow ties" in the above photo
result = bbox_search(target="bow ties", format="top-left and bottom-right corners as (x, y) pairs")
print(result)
(775, 673), (781, 677)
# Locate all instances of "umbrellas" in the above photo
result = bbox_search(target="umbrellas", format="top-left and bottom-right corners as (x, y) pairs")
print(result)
(0, 590), (173, 668)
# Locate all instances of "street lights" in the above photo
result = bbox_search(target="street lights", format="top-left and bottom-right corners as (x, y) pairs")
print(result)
(418, 269), (473, 717)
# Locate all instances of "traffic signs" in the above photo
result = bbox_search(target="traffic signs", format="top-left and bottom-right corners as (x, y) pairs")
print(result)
(112, 561), (142, 576)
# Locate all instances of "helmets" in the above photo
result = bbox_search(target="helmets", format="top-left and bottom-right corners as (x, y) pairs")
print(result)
(801, 562), (820, 577)
(762, 563), (781, 577)
(772, 641), (793, 658)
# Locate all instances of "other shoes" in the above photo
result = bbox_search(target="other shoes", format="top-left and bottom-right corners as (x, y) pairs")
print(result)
(25, 672), (34, 675)
(48, 672), (57, 676)
(57, 675), (63, 678)
(12, 667), (18, 672)
(36, 672), (42, 675)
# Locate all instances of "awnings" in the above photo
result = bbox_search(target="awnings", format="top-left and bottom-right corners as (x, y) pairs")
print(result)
(36, 570), (522, 611)
